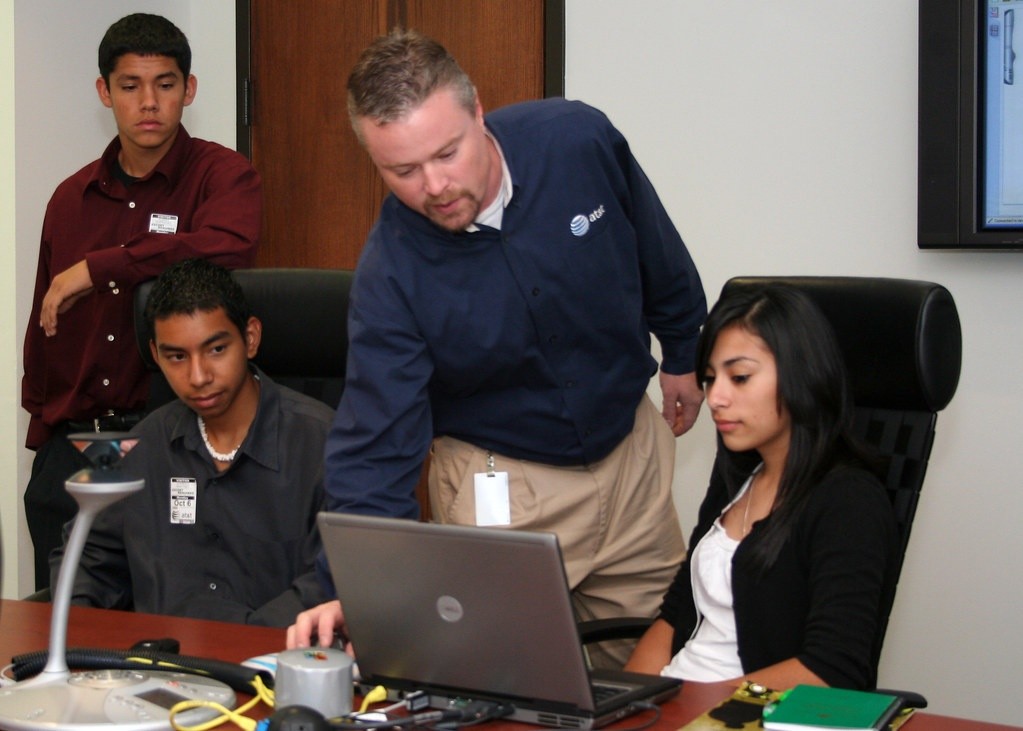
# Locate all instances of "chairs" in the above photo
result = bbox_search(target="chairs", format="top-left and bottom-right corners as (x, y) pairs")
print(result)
(573, 275), (963, 688)
(134, 268), (358, 413)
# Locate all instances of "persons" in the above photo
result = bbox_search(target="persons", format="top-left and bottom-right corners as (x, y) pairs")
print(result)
(49, 259), (335, 632)
(622, 284), (894, 693)
(284, 25), (709, 676)
(21, 14), (266, 597)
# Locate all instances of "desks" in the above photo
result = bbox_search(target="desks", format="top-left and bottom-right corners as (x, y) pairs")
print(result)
(1, 600), (1023, 731)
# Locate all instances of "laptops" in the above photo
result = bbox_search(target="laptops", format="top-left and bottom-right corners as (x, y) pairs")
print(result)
(316, 510), (684, 731)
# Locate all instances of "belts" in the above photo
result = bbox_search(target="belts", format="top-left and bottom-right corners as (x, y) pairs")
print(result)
(63, 413), (142, 433)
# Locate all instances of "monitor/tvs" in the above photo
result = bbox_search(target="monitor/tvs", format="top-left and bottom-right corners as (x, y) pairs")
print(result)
(918, 0), (1023, 250)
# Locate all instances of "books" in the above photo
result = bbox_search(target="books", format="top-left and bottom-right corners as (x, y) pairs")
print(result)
(762, 684), (903, 731)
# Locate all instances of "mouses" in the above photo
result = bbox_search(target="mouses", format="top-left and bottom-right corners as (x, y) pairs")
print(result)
(311, 628), (349, 653)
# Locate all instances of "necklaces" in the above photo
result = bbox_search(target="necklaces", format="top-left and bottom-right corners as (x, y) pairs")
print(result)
(196, 415), (237, 462)
(743, 470), (761, 536)
